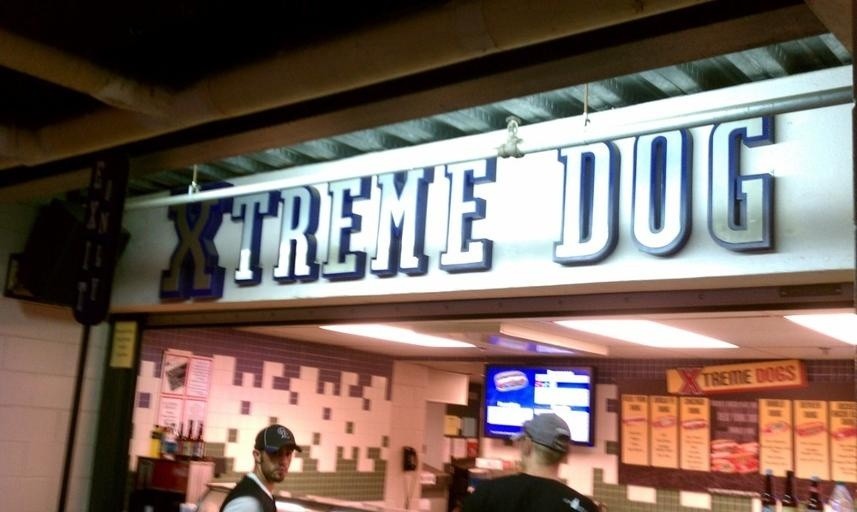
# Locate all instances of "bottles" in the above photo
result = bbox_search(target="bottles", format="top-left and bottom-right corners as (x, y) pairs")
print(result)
(761, 468), (824, 512)
(149, 420), (206, 459)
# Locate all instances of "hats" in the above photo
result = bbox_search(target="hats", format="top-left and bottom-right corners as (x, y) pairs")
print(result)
(254, 424), (303, 454)
(510, 413), (570, 454)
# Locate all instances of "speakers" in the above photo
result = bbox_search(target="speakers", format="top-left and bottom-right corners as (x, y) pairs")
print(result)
(18, 200), (132, 307)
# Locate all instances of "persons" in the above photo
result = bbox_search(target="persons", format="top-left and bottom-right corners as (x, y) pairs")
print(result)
(460, 413), (601, 512)
(218, 424), (302, 512)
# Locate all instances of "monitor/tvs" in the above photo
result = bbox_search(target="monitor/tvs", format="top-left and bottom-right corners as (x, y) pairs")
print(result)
(482, 362), (595, 447)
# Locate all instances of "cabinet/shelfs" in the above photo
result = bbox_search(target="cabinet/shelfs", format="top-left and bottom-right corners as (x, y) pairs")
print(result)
(134, 456), (215, 512)
